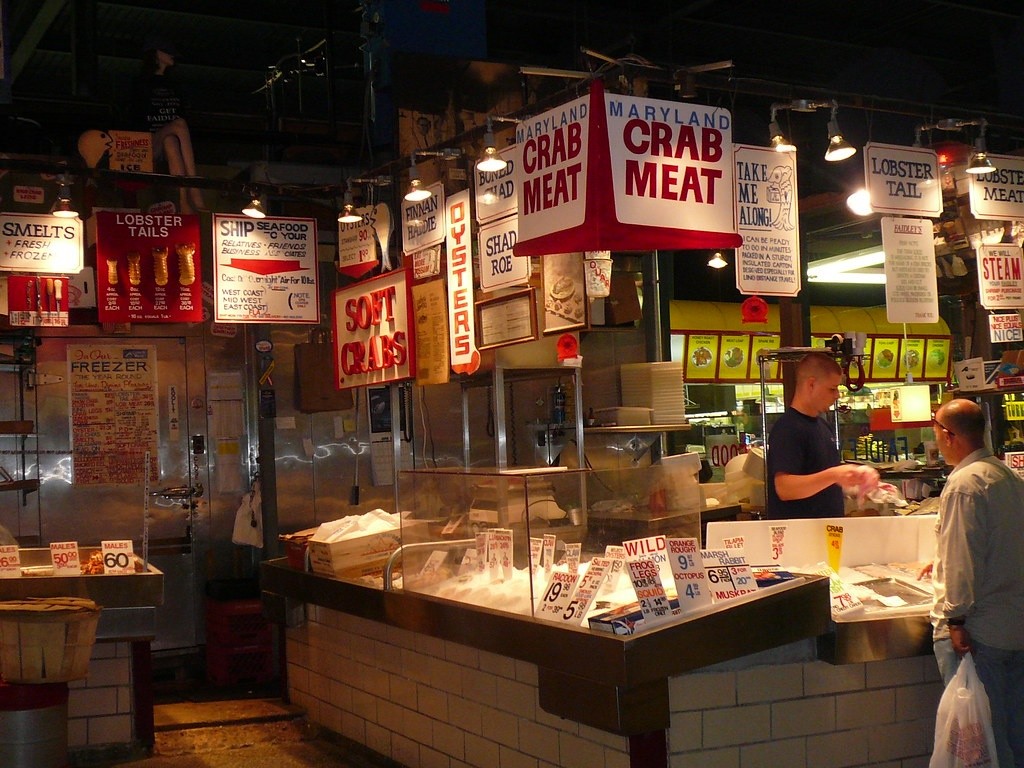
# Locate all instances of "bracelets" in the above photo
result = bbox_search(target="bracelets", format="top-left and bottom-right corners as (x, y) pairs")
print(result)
(946, 617), (966, 626)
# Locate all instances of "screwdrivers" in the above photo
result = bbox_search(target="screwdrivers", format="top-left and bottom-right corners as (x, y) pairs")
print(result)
(46, 279), (53, 322)
(54, 279), (62, 320)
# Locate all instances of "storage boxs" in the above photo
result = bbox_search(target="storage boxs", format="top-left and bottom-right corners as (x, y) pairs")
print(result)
(589, 270), (643, 326)
(205, 593), (280, 690)
(277, 510), (429, 577)
(831, 563), (934, 665)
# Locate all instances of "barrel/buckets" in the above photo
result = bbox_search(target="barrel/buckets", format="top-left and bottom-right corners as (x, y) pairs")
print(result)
(0, 678), (69, 768)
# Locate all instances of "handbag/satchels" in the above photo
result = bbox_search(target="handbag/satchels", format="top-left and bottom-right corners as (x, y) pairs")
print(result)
(928, 652), (1001, 767)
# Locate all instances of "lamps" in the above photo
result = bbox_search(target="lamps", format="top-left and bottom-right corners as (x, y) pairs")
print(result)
(338, 175), (392, 223)
(912, 109), (996, 173)
(404, 150), (462, 201)
(707, 248), (729, 268)
(815, 97), (856, 161)
(241, 177), (274, 218)
(475, 115), (526, 172)
(49, 169), (80, 218)
(890, 323), (932, 423)
(846, 96), (876, 217)
(760, 96), (815, 153)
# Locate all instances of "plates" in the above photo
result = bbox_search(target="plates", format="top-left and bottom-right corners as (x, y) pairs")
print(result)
(876, 349), (895, 369)
(754, 347), (773, 367)
(691, 347), (713, 368)
(927, 350), (945, 369)
(723, 347), (745, 369)
(902, 349), (921, 369)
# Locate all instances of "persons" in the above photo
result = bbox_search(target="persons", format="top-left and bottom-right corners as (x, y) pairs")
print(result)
(928, 397), (1024, 768)
(766, 352), (881, 521)
(231, 458), (319, 692)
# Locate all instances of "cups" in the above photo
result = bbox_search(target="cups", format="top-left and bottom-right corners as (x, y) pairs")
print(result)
(567, 508), (582, 526)
(1009, 430), (1020, 440)
(923, 441), (940, 467)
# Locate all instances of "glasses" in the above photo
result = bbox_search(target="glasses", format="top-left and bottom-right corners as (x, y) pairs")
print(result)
(932, 413), (955, 436)
